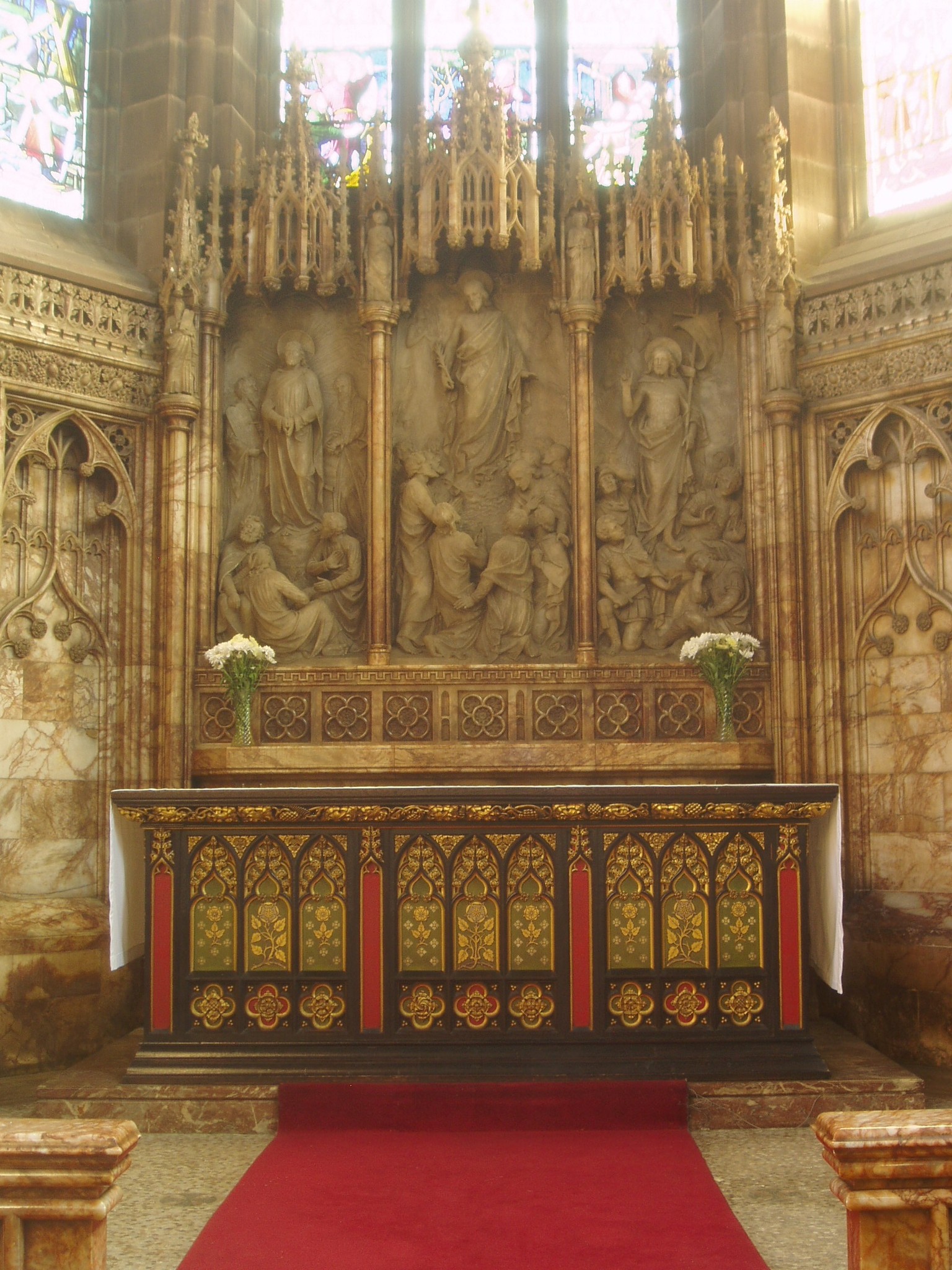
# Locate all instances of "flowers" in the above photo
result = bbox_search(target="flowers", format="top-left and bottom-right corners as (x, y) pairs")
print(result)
(679, 631), (760, 685)
(204, 634), (277, 696)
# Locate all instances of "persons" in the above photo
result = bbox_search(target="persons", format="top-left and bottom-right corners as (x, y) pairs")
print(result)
(392, 279), (570, 657)
(597, 346), (747, 656)
(217, 342), (364, 657)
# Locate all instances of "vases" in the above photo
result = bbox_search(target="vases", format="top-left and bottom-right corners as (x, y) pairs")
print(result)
(713, 687), (738, 742)
(231, 689), (256, 746)
